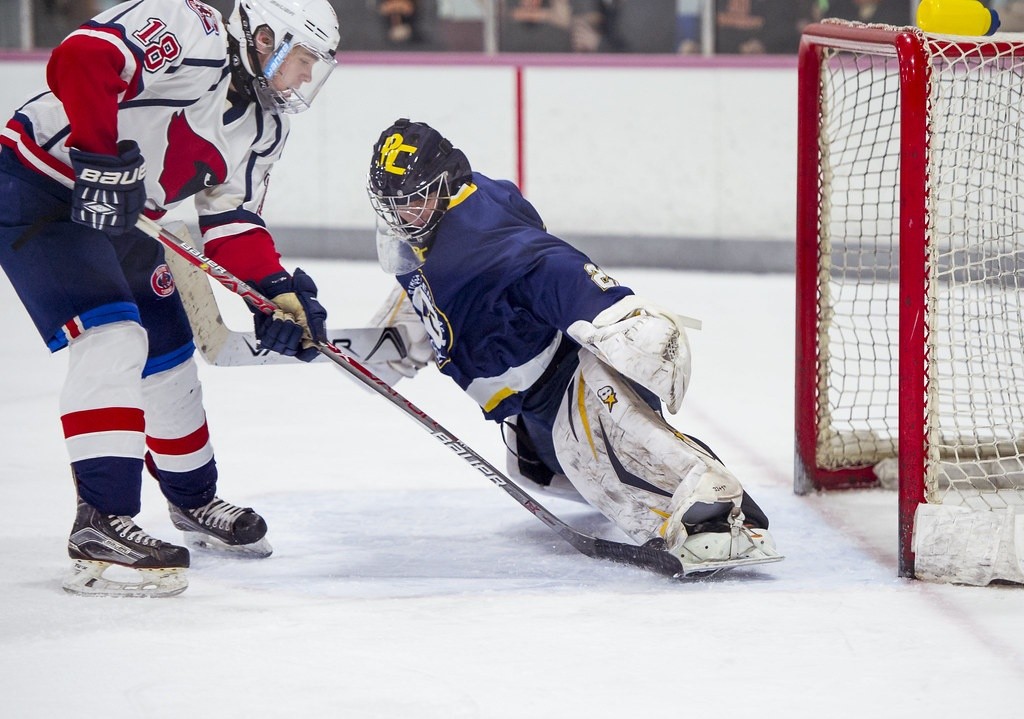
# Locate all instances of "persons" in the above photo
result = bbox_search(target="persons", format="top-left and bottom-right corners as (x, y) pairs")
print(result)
(0, 0), (340, 598)
(347, 119), (786, 573)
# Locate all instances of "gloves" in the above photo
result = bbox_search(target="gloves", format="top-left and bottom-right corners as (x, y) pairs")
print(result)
(242, 267), (327, 363)
(594, 312), (690, 415)
(67, 140), (147, 234)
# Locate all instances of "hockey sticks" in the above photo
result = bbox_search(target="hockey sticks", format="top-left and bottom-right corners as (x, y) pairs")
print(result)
(133, 215), (685, 580)
(157, 221), (413, 367)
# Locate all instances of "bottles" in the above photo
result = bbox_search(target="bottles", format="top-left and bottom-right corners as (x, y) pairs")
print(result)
(916, 0), (1001, 36)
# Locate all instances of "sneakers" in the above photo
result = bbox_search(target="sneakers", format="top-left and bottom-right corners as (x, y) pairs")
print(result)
(670, 524), (786, 575)
(62, 495), (191, 599)
(168, 495), (273, 558)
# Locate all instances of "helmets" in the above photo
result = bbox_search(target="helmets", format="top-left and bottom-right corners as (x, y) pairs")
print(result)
(365, 118), (474, 245)
(227, 0), (340, 94)
